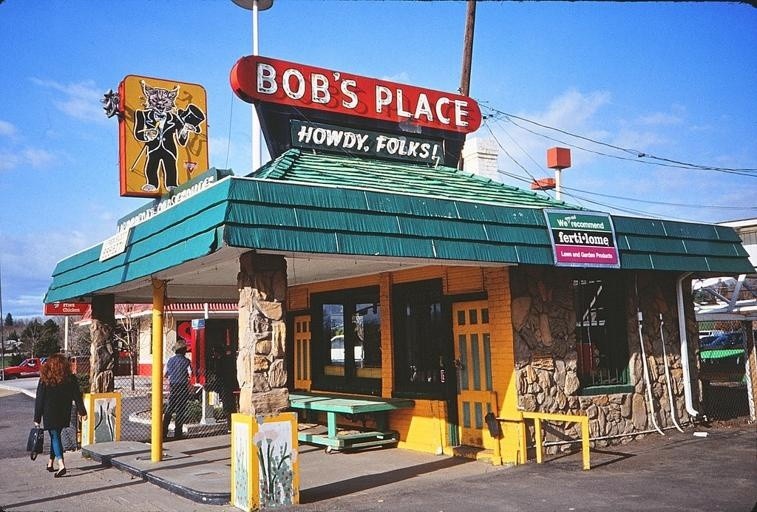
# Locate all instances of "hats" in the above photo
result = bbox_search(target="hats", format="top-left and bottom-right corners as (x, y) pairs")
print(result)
(175, 340), (187, 350)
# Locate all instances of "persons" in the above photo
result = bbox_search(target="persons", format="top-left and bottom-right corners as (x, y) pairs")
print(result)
(130, 81), (193, 193)
(163, 339), (192, 440)
(31, 352), (86, 478)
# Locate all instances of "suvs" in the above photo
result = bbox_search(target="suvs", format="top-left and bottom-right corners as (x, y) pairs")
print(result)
(330, 335), (364, 363)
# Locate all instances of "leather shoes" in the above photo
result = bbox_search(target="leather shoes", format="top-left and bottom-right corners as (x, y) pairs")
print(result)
(47, 464), (54, 472)
(54, 468), (66, 478)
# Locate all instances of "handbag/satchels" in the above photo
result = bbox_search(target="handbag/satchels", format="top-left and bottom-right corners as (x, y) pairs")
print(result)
(26, 426), (44, 461)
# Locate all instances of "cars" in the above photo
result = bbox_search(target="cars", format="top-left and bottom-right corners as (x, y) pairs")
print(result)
(0, 357), (48, 380)
(697, 329), (756, 373)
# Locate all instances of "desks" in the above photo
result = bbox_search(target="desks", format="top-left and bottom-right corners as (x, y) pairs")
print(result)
(288, 391), (415, 453)
